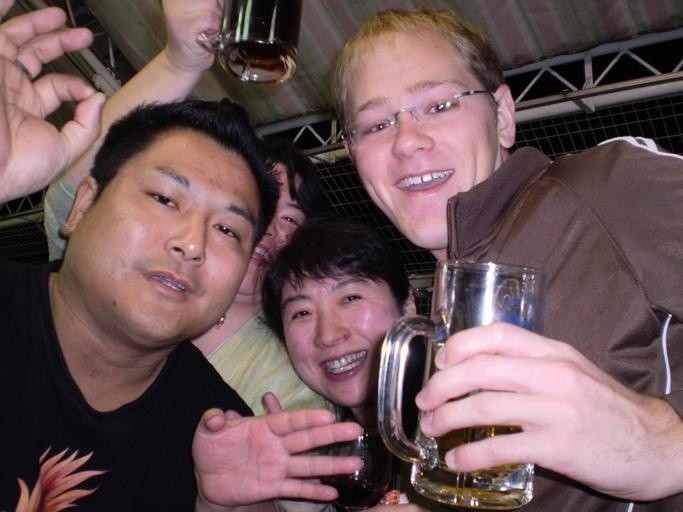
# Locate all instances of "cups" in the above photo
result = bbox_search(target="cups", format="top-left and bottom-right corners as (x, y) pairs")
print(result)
(195, 0), (304, 86)
(377, 259), (546, 508)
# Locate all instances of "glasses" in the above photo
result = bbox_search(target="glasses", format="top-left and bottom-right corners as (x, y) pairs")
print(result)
(340, 89), (498, 146)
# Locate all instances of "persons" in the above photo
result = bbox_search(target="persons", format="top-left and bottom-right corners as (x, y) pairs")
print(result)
(328, 5), (683, 512)
(42, 0), (356, 458)
(258, 217), (417, 511)
(0, 0), (366, 512)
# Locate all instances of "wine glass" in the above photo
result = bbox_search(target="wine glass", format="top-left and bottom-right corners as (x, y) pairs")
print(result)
(321, 430), (392, 512)
